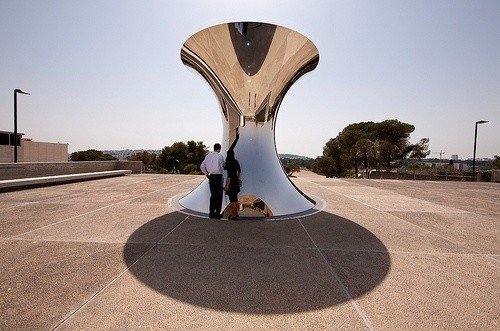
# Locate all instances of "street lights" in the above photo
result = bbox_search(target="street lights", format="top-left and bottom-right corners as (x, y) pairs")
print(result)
(472, 120), (488, 182)
(12, 88), (29, 162)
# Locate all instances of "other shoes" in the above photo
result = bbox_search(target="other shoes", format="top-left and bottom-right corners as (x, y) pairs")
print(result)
(216, 214), (223, 219)
(209, 214), (216, 218)
(228, 214), (239, 221)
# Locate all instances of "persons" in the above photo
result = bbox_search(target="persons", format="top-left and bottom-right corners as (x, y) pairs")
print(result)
(224, 149), (241, 221)
(200, 143), (226, 219)
